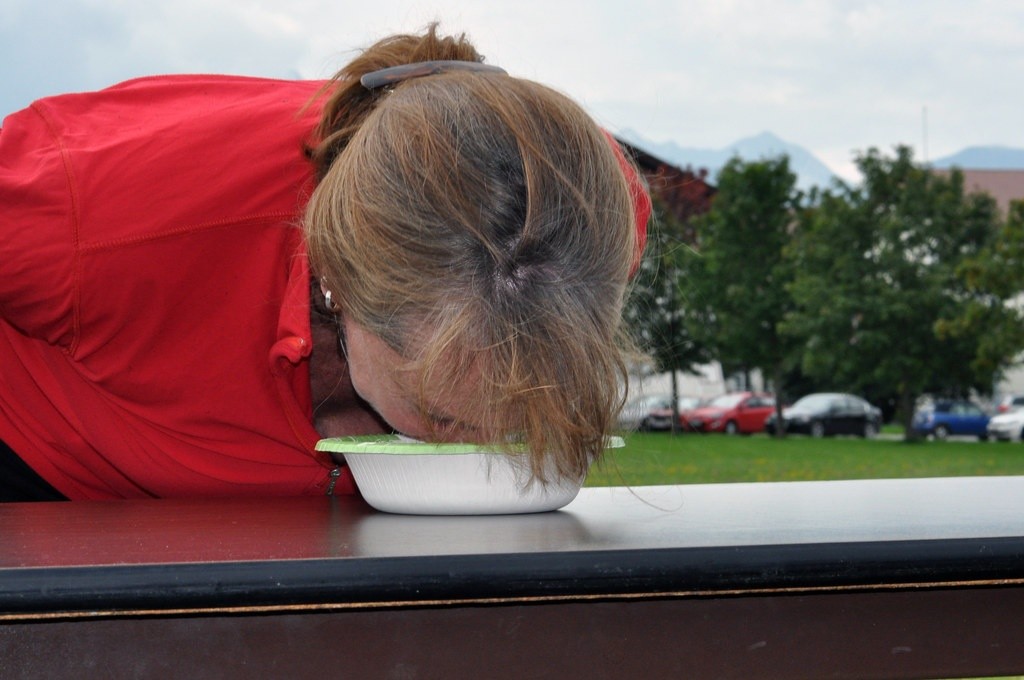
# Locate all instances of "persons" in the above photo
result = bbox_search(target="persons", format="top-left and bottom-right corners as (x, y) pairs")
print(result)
(1, 23), (654, 500)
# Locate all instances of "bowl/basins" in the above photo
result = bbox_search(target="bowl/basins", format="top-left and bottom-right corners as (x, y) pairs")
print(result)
(314, 429), (624, 514)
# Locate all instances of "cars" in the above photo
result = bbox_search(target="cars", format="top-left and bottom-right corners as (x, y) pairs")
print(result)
(764, 392), (883, 440)
(986, 396), (1024, 443)
(681, 389), (789, 437)
(613, 395), (670, 432)
(642, 397), (706, 433)
(912, 397), (992, 441)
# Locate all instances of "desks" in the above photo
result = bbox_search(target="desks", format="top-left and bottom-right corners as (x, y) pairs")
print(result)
(0, 475), (1024, 680)
